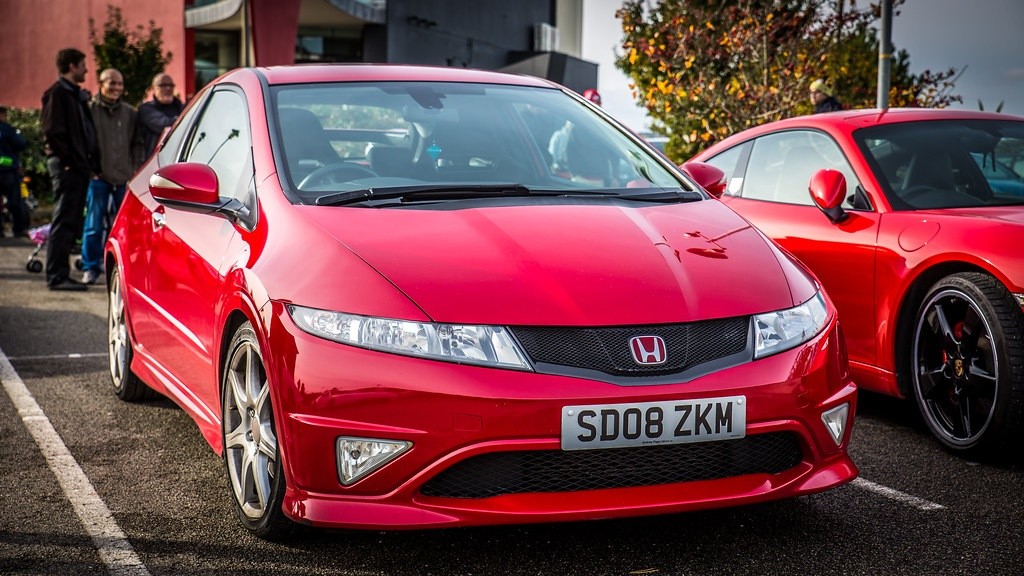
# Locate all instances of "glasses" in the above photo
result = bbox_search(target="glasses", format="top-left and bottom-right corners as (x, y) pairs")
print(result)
(809, 91), (818, 94)
(153, 83), (175, 88)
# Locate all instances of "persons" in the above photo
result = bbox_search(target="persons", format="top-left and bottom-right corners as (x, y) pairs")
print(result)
(522, 90), (620, 186)
(40, 48), (102, 290)
(137, 73), (186, 165)
(810, 78), (842, 115)
(82, 68), (136, 282)
(0, 107), (33, 237)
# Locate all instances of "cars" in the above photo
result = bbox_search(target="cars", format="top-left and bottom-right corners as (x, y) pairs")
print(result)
(623, 106), (1020, 463)
(98, 59), (861, 539)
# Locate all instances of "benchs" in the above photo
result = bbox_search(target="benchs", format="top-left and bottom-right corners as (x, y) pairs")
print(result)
(364, 142), (435, 177)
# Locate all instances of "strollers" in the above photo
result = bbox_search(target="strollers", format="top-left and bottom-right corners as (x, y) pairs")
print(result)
(23, 214), (102, 278)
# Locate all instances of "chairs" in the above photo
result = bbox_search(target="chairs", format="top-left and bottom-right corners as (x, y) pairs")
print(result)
(273, 106), (359, 185)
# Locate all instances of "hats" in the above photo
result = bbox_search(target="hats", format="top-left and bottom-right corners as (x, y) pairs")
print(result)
(583, 88), (601, 103)
(809, 76), (833, 97)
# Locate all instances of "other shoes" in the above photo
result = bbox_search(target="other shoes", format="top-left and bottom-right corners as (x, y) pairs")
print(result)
(48, 277), (90, 290)
(13, 230), (29, 238)
(81, 270), (97, 284)
